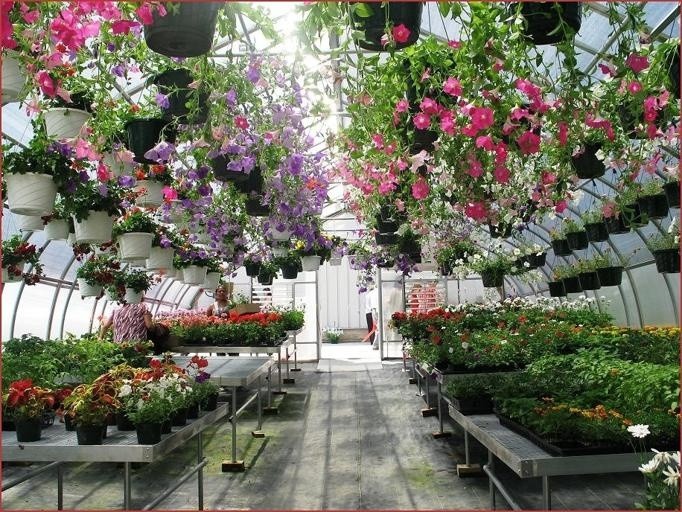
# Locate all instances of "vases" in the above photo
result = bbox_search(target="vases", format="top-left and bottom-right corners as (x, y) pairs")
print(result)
(1, 411), (186, 447)
(186, 392), (218, 420)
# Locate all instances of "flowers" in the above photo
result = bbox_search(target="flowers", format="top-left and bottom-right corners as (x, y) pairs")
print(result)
(532, 392), (681, 487)
(88, 296), (305, 347)
(1, 332), (212, 420)
(321, 321), (344, 343)
(392, 291), (680, 364)
(300, 1), (682, 294)
(2, 0), (330, 304)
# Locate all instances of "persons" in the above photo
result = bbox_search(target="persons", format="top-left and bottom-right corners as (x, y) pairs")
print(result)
(205, 285), (240, 356)
(365, 286), (378, 344)
(96, 292), (155, 343)
